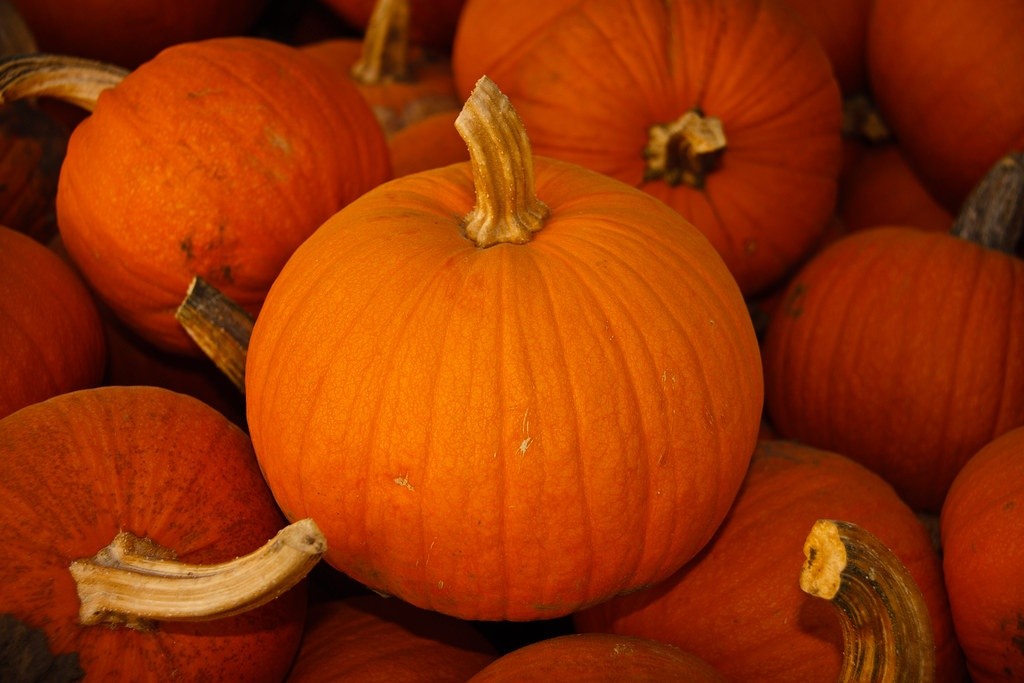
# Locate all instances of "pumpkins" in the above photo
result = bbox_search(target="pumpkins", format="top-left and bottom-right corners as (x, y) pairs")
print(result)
(0, 0), (1024, 683)
